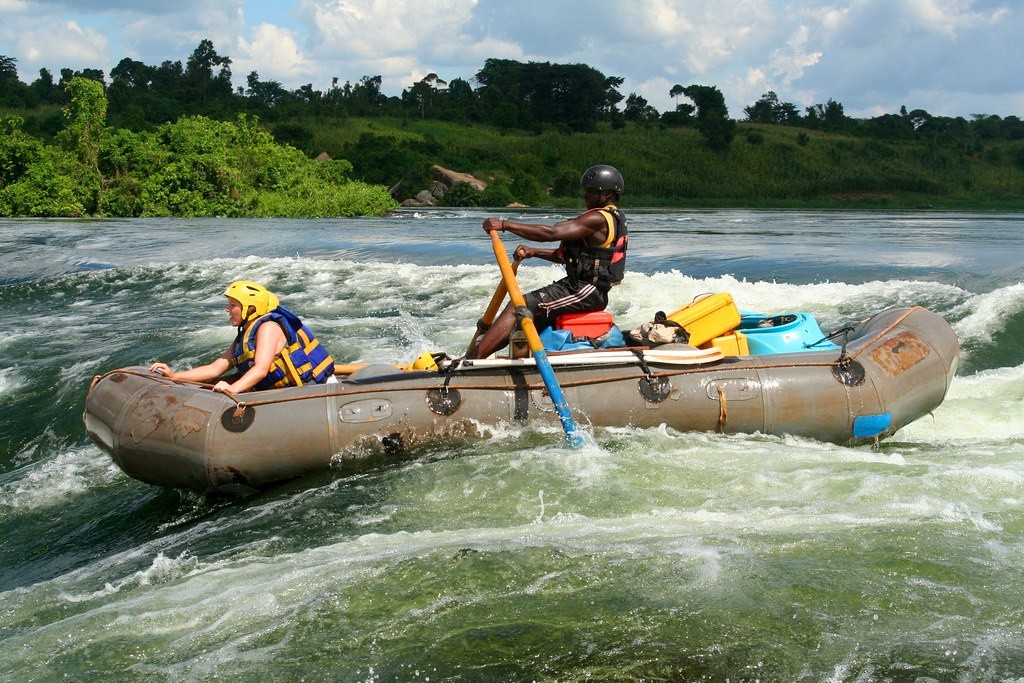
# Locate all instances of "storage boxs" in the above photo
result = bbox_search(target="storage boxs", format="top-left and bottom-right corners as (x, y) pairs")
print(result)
(667, 293), (742, 347)
(557, 310), (614, 338)
(700, 330), (750, 357)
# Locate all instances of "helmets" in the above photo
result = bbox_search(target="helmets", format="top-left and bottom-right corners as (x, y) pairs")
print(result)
(580, 165), (626, 195)
(411, 352), (439, 371)
(269, 291), (278, 312)
(224, 280), (270, 316)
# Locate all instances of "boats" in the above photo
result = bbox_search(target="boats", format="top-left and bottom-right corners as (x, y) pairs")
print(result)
(81, 302), (958, 501)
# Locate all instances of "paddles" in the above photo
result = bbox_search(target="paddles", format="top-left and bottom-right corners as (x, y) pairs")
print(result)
(464, 245), (526, 361)
(489, 227), (584, 448)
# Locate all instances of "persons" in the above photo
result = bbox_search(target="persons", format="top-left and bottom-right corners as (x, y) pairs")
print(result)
(150, 281), (337, 393)
(461, 164), (628, 360)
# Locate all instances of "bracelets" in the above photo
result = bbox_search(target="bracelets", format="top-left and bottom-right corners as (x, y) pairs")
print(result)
(502, 220), (506, 232)
